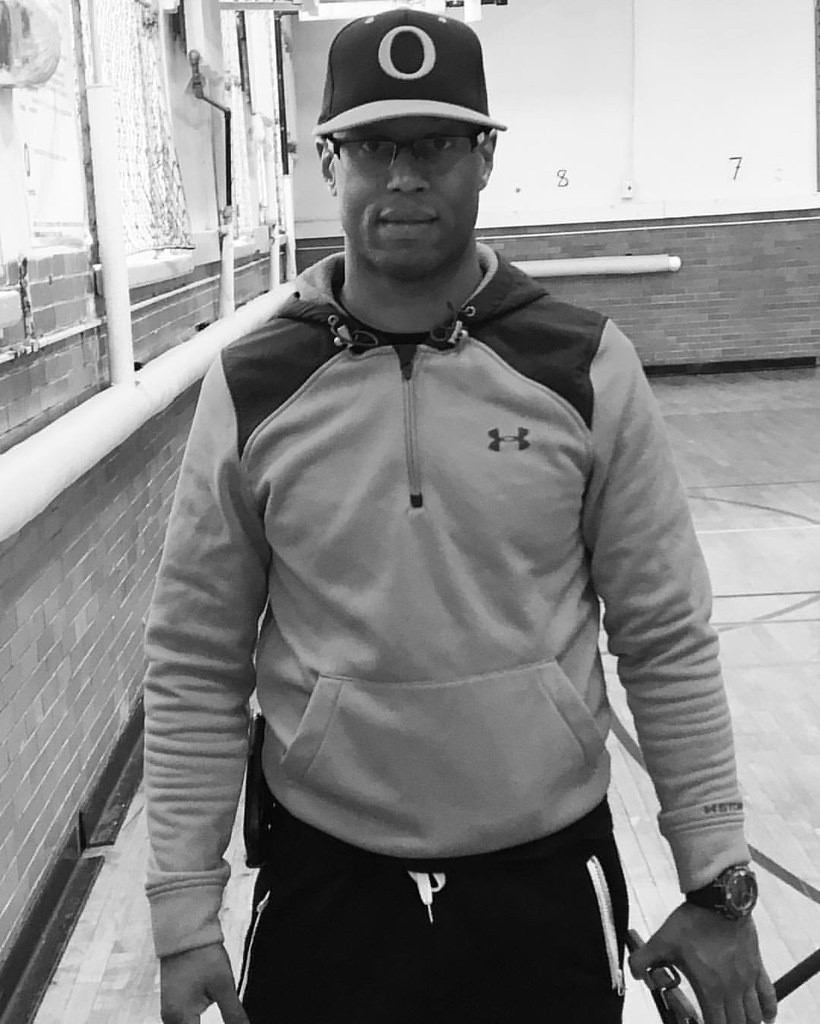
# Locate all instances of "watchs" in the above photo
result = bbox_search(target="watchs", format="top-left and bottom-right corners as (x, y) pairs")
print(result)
(686, 865), (758, 920)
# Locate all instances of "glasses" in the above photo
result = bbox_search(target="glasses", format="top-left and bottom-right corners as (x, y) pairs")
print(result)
(324, 132), (480, 174)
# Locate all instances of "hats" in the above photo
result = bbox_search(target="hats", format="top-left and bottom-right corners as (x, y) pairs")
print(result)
(312, 8), (507, 145)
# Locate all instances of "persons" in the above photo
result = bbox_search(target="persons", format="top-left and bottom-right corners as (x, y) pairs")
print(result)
(145, 7), (778, 1024)
